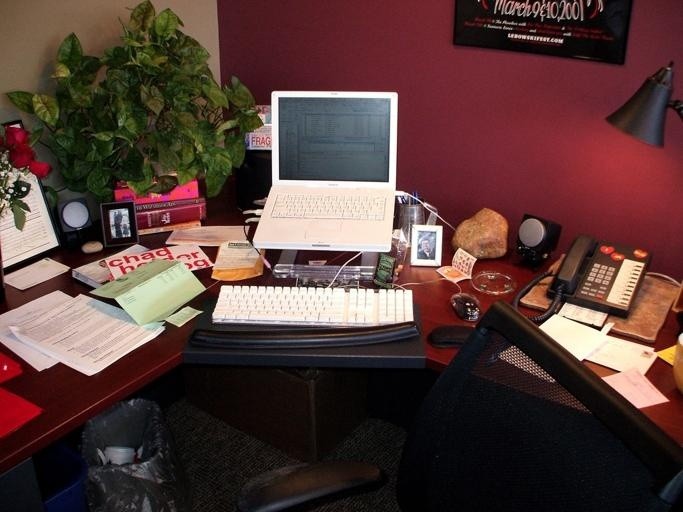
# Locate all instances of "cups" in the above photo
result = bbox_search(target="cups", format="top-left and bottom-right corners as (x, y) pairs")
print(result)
(397, 203), (427, 247)
(373, 242), (407, 288)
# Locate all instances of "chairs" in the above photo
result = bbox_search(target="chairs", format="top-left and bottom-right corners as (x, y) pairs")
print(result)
(234, 302), (682, 512)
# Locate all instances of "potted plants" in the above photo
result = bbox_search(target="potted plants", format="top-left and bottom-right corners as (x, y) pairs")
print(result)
(6, 0), (263, 237)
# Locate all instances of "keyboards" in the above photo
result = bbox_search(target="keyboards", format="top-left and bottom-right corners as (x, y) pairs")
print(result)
(212, 284), (415, 329)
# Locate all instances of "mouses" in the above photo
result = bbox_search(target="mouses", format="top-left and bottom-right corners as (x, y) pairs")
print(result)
(451, 291), (483, 321)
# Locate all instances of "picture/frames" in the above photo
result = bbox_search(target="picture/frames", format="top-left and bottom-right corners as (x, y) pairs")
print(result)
(453, 0), (633, 65)
(0, 120), (65, 272)
(410, 224), (443, 268)
(99, 200), (140, 249)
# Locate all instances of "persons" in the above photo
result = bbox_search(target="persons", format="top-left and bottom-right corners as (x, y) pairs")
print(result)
(114, 214), (129, 238)
(417, 239), (434, 259)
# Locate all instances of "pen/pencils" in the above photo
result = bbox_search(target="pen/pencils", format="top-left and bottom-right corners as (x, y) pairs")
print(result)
(397, 191), (420, 206)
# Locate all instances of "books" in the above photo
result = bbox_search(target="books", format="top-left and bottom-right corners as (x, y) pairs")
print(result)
(113, 177), (208, 236)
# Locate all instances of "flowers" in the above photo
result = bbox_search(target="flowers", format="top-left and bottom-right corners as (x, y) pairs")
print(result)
(0, 125), (52, 231)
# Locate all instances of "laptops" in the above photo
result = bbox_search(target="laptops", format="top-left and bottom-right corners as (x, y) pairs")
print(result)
(252, 90), (399, 253)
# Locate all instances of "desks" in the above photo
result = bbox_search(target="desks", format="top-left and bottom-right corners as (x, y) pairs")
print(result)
(1, 206), (683, 486)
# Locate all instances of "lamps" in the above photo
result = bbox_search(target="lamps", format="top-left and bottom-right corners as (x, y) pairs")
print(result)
(605, 59), (683, 148)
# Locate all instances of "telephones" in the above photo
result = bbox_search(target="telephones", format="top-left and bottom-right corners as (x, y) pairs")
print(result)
(512, 234), (653, 323)
